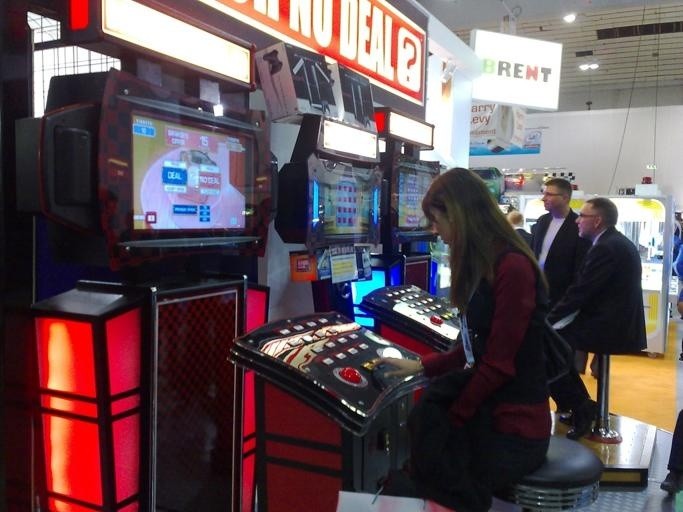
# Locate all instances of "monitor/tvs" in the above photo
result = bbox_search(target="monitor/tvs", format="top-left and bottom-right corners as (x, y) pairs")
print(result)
(305, 150), (386, 249)
(127, 103), (256, 246)
(394, 162), (439, 235)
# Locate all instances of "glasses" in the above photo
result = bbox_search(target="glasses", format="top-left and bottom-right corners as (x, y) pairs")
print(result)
(578, 213), (598, 218)
(543, 192), (566, 197)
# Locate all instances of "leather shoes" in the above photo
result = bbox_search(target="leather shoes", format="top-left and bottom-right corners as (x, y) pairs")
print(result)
(660, 472), (683, 494)
(559, 411), (572, 423)
(566, 400), (598, 439)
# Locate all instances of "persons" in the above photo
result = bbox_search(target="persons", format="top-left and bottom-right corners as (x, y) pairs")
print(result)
(548, 197), (647, 439)
(372, 168), (554, 506)
(526, 177), (588, 308)
(660, 404), (678, 492)
(505, 209), (533, 246)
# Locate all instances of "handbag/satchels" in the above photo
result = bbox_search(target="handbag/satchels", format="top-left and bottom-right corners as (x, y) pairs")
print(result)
(489, 247), (578, 406)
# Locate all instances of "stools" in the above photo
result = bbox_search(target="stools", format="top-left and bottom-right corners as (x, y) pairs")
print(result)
(571, 337), (644, 445)
(495, 431), (603, 512)
